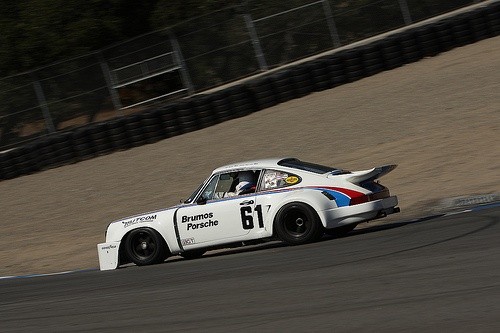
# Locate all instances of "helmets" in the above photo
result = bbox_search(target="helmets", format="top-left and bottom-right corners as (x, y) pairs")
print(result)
(234, 171), (254, 191)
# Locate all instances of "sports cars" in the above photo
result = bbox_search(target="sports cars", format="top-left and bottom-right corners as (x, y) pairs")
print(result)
(96, 158), (399, 271)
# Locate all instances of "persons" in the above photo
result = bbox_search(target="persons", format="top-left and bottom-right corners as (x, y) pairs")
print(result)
(203, 170), (258, 200)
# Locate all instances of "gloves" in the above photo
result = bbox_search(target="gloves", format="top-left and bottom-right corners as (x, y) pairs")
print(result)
(202, 190), (226, 199)
